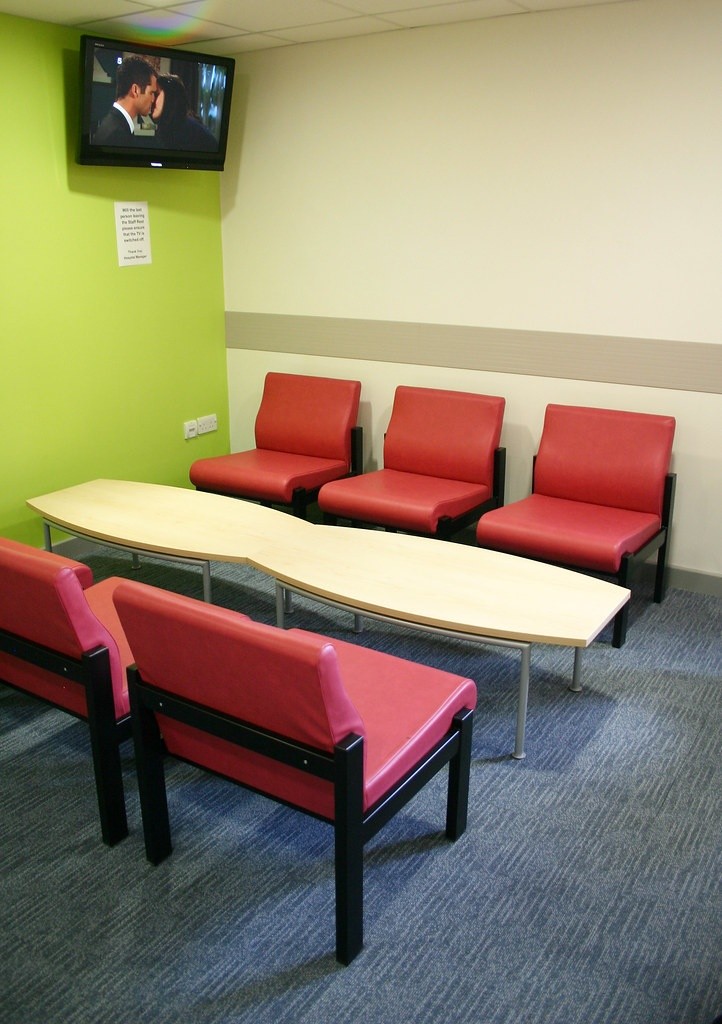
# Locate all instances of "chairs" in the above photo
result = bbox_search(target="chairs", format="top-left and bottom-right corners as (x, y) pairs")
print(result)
(190, 372), (363, 533)
(318, 385), (506, 544)
(0, 536), (93, 593)
(112, 581), (479, 968)
(475, 404), (677, 649)
(1, 537), (253, 849)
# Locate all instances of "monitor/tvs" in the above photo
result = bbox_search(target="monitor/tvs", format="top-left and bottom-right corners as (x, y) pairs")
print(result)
(75, 33), (235, 171)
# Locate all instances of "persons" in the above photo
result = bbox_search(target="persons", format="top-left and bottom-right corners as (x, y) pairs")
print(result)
(150, 72), (219, 153)
(91, 55), (160, 148)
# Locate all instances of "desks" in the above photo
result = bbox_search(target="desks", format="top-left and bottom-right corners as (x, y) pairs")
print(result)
(26, 476), (632, 759)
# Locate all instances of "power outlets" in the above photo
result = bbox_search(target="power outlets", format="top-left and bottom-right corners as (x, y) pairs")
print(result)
(196, 414), (218, 434)
(184, 420), (197, 440)
